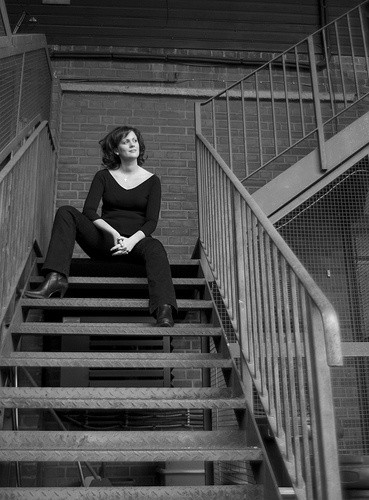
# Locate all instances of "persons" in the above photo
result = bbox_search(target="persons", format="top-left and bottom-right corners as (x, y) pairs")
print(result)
(24, 126), (179, 327)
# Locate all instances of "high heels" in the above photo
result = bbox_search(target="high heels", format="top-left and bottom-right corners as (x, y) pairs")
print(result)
(155, 304), (175, 327)
(25, 271), (68, 299)
